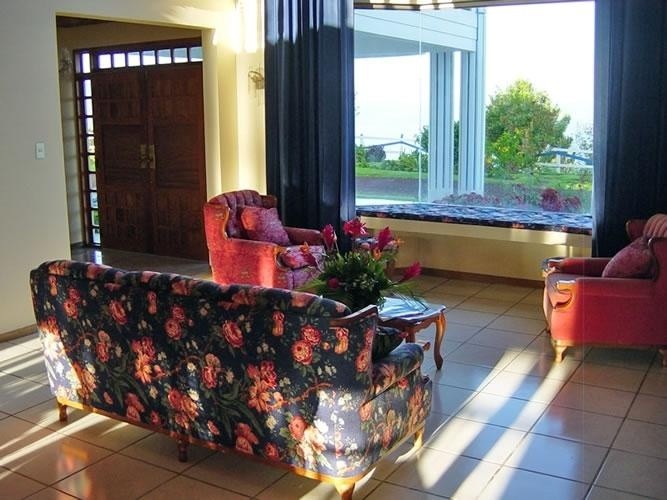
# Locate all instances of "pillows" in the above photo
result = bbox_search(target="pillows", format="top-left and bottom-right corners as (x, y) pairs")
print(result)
(599, 236), (652, 279)
(234, 202), (288, 243)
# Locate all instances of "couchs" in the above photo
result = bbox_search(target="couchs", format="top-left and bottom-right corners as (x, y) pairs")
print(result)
(29, 257), (437, 499)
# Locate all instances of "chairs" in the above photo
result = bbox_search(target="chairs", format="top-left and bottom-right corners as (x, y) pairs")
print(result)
(538, 214), (666, 372)
(202, 189), (328, 297)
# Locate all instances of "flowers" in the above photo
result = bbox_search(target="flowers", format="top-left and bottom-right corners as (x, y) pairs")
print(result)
(295, 220), (422, 306)
